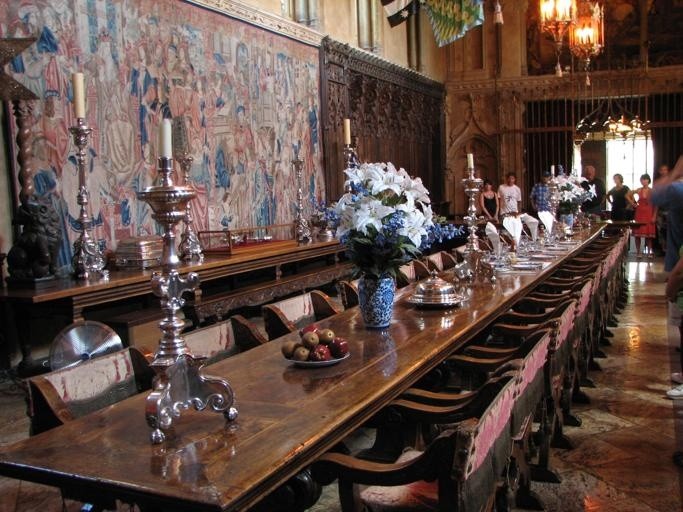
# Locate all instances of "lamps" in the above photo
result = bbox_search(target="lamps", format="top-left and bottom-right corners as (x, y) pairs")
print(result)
(539, 0), (572, 77)
(569, 1), (608, 86)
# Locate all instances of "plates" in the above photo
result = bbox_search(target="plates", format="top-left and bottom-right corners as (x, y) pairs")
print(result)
(512, 241), (578, 270)
(283, 352), (351, 367)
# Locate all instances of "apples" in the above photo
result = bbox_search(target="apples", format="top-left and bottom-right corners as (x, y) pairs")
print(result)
(331, 337), (349, 358)
(299, 323), (321, 337)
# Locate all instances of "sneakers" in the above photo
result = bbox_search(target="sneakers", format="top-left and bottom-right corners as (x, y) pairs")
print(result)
(637, 253), (654, 259)
(666, 384), (683, 400)
(671, 372), (683, 383)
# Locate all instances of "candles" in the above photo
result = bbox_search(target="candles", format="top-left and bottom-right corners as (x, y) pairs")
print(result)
(550, 165), (556, 174)
(466, 152), (474, 168)
(158, 119), (174, 159)
(342, 117), (353, 146)
(73, 74), (87, 119)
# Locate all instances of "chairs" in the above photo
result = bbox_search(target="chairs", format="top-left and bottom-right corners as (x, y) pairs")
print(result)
(1, 210), (632, 512)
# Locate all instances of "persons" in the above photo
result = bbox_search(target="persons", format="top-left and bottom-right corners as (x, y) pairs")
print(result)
(606, 174), (658, 258)
(653, 153), (683, 397)
(580, 166), (606, 213)
(530, 172), (551, 212)
(480, 173), (522, 223)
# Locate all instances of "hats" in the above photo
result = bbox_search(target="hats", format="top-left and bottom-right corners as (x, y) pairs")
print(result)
(543, 171), (551, 176)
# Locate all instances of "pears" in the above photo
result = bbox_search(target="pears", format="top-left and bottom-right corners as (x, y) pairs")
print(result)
(281, 328), (335, 362)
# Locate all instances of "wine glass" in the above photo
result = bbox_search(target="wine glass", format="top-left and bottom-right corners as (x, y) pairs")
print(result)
(494, 242), (503, 269)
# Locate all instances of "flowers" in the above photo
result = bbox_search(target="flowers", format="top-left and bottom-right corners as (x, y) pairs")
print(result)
(312, 159), (427, 269)
